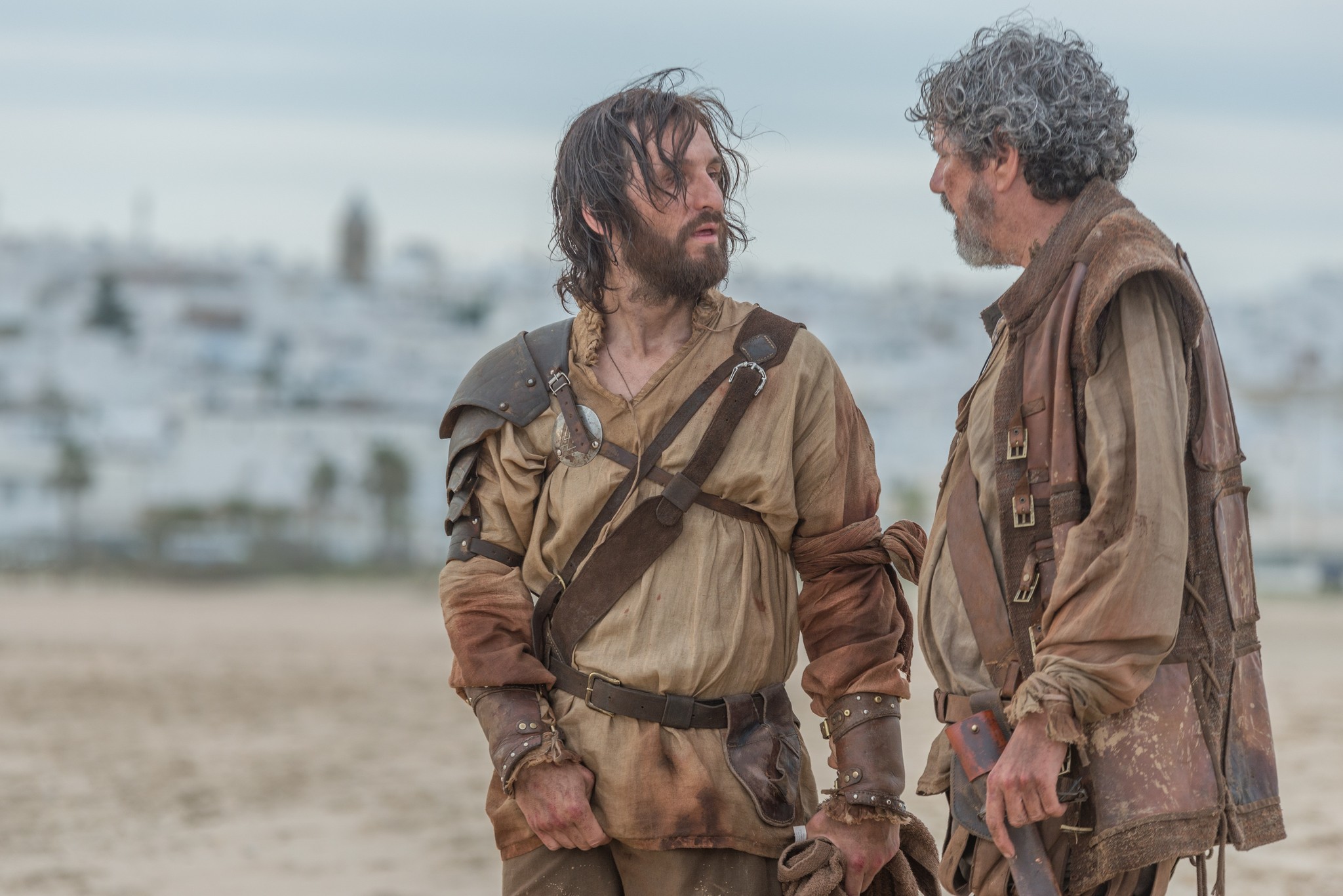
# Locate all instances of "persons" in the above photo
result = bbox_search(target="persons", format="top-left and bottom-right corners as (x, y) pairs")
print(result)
(438, 64), (929, 896)
(918, 18), (1286, 896)
